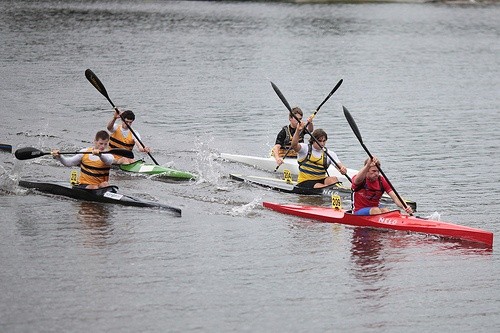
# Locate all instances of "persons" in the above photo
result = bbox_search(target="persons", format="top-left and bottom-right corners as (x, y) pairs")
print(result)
(51, 130), (114, 195)
(107, 108), (150, 165)
(351, 157), (413, 215)
(271, 107), (314, 164)
(291, 121), (347, 188)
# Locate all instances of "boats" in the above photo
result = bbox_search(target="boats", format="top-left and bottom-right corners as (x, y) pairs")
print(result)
(72, 155), (193, 183)
(18, 180), (182, 222)
(222, 153), (493, 248)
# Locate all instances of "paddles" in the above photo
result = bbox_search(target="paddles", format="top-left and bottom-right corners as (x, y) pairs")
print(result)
(273, 78), (352, 183)
(84, 68), (160, 165)
(268, 78), (345, 172)
(14, 147), (134, 162)
(342, 105), (414, 215)
